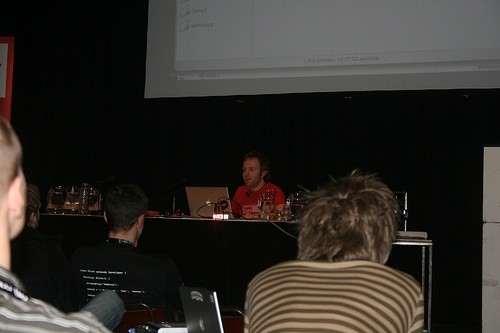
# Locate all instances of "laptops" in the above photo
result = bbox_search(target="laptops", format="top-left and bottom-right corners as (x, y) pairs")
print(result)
(185, 186), (237, 219)
(178, 287), (225, 333)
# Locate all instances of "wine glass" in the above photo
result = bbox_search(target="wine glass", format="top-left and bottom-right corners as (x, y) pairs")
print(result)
(50, 183), (103, 214)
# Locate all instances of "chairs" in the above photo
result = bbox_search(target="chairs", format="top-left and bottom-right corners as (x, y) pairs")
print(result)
(391, 191), (433, 332)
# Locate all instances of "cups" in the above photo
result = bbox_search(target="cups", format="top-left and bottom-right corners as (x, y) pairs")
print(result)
(392, 191), (408, 231)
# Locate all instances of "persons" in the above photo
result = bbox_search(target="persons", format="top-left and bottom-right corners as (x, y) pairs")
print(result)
(10, 179), (86, 313)
(243, 172), (424, 333)
(0, 114), (125, 333)
(230, 153), (285, 219)
(78, 180), (181, 322)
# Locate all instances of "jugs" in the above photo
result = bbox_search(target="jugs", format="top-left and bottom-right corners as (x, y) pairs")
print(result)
(285, 192), (305, 221)
(257, 189), (277, 220)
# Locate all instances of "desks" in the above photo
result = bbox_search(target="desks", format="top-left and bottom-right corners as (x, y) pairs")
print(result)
(37, 213), (298, 316)
